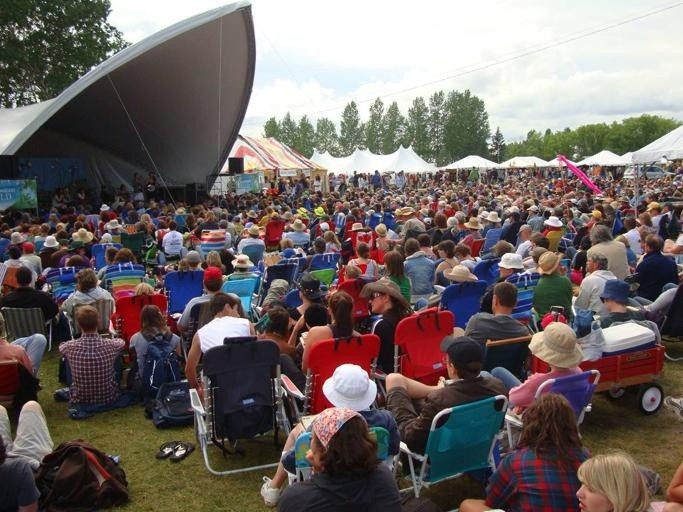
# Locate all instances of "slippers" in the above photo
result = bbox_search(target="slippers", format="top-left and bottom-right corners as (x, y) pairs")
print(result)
(155, 440), (196, 461)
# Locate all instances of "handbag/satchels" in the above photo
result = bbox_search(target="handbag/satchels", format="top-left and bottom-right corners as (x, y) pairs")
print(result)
(33, 438), (129, 511)
(152, 378), (206, 430)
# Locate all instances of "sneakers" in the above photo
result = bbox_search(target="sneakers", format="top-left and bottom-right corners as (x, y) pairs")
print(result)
(260, 480), (283, 508)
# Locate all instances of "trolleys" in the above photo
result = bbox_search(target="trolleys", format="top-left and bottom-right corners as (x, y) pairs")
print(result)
(525, 320), (664, 415)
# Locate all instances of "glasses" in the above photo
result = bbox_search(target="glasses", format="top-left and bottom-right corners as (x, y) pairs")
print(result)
(600, 297), (606, 303)
(369, 292), (385, 301)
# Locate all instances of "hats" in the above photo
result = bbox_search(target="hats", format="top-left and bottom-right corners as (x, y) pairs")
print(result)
(527, 321), (585, 368)
(358, 278), (409, 313)
(599, 279), (631, 307)
(322, 363), (378, 412)
(292, 272), (321, 300)
(438, 334), (483, 368)
(204, 266), (223, 281)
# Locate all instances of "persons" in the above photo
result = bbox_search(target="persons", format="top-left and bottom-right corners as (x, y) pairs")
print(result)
(0, 160), (683, 512)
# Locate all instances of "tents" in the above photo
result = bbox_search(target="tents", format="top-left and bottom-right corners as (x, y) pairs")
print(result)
(577, 150), (632, 167)
(211, 138), (440, 196)
(442, 154), (499, 169)
(547, 156), (577, 168)
(632, 125), (683, 218)
(497, 155), (550, 168)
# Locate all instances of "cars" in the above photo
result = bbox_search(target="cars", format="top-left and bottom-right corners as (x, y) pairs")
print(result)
(622, 164), (677, 181)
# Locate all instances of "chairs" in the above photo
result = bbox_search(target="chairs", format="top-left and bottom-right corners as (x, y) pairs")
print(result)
(0, 361), (35, 424)
(281, 334), (381, 428)
(1, 307), (53, 353)
(284, 427), (390, 485)
(392, 395), (509, 499)
(65, 298), (114, 341)
(189, 340), (293, 475)
(91, 220), (683, 361)
(481, 336), (534, 379)
(41, 267), (85, 307)
(505, 369), (602, 449)
(376, 310), (455, 404)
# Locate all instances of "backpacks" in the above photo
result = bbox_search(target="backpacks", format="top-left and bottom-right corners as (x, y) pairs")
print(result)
(139, 329), (181, 396)
